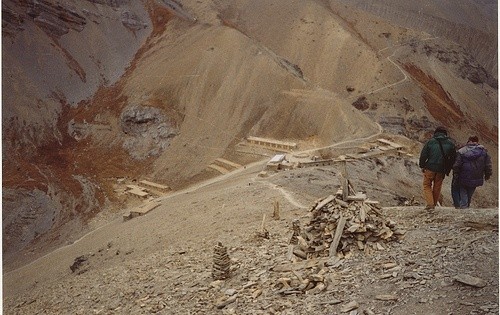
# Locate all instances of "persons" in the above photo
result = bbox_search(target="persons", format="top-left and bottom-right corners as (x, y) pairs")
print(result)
(418, 126), (457, 211)
(450, 136), (493, 210)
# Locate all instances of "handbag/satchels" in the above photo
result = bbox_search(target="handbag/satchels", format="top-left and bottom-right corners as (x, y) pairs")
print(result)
(446, 156), (454, 175)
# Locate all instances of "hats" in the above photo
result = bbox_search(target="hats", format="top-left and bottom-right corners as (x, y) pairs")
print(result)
(434, 126), (447, 135)
(468, 135), (478, 141)
